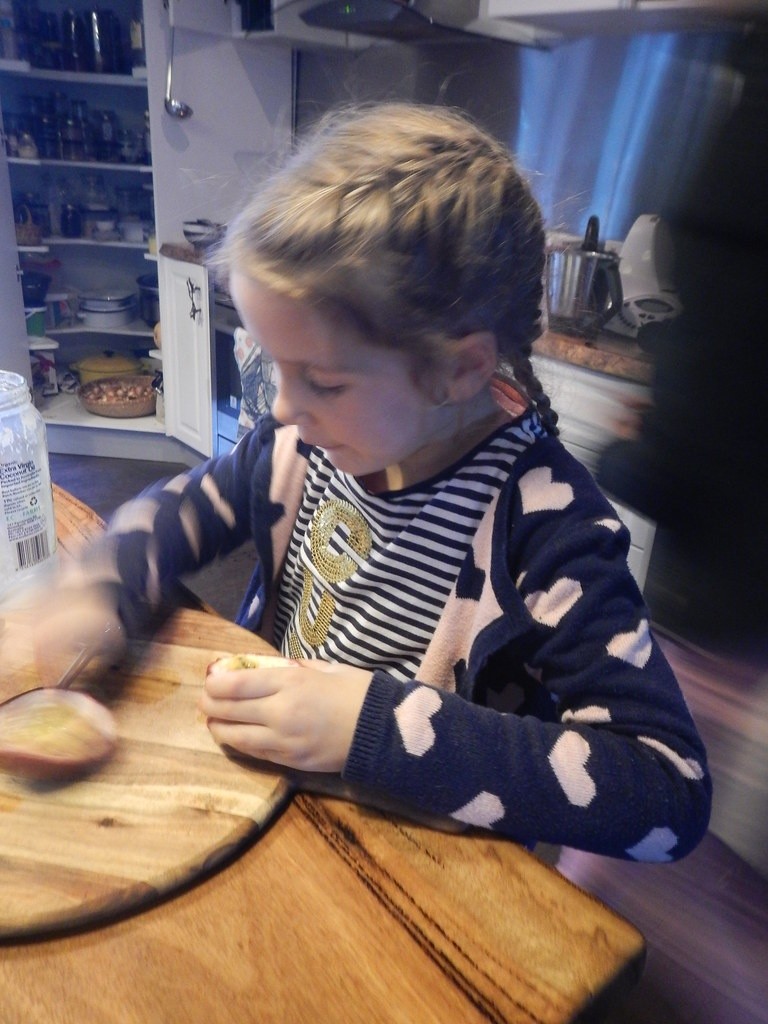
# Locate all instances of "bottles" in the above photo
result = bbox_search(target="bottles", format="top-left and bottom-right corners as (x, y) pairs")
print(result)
(0, 1), (152, 174)
(0, 370), (61, 584)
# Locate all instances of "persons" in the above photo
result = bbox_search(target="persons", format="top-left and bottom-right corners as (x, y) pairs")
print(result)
(38, 104), (712, 866)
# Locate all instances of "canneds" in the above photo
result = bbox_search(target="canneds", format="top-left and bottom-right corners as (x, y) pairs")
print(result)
(60, 202), (82, 238)
(26, 88), (138, 162)
(0, 0), (124, 76)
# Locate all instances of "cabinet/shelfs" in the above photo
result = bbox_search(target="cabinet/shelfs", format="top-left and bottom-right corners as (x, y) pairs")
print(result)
(0, 0), (766, 593)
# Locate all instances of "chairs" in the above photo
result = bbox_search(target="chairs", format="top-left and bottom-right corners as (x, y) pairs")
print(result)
(536, 485), (658, 868)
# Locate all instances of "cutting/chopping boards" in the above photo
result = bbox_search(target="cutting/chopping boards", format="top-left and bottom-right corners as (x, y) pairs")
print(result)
(0, 596), (314, 940)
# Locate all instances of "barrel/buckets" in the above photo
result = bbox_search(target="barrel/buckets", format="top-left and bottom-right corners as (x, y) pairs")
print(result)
(24, 306), (49, 339)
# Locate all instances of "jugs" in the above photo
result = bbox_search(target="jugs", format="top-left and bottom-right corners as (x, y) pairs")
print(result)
(542, 215), (623, 347)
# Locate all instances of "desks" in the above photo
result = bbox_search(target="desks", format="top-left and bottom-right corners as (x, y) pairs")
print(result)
(0, 481), (648, 1024)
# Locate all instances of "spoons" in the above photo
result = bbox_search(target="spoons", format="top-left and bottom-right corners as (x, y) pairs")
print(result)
(160, 1), (194, 121)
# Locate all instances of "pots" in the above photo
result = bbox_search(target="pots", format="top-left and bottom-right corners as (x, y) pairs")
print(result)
(69, 348), (143, 386)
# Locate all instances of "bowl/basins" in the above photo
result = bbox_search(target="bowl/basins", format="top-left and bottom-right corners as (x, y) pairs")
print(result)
(20, 269), (52, 305)
